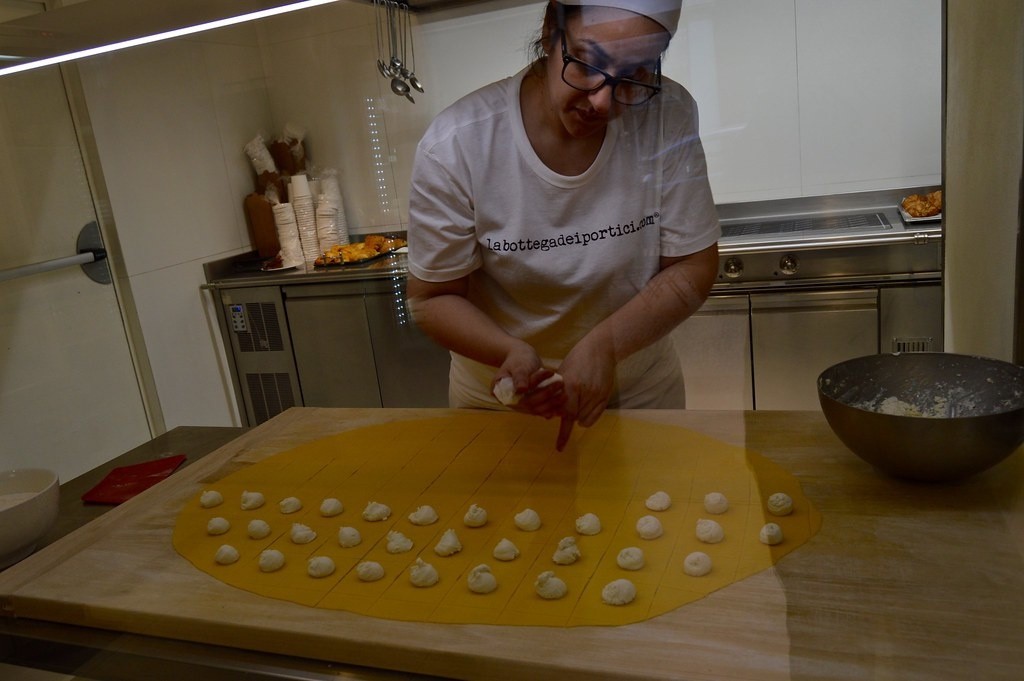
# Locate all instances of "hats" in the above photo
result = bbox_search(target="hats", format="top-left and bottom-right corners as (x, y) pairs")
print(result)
(557, 0), (682, 39)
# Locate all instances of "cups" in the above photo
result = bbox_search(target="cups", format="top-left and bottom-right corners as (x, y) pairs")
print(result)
(273, 176), (349, 263)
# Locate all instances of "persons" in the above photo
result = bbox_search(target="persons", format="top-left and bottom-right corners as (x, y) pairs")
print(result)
(404, 0), (724, 452)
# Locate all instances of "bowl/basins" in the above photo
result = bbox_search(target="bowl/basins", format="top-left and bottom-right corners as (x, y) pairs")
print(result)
(817, 351), (1024, 480)
(0, 468), (61, 556)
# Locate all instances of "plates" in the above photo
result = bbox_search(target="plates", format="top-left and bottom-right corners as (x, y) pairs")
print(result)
(261, 263), (298, 271)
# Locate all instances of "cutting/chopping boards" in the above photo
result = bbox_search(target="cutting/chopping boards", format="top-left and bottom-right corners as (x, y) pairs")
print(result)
(0, 406), (1024, 681)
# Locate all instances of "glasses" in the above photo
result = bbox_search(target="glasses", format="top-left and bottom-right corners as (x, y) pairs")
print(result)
(557, 2), (662, 106)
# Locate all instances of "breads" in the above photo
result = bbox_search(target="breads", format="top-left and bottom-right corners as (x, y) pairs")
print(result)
(316, 236), (404, 264)
